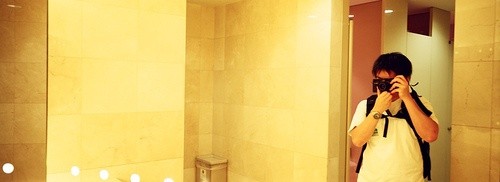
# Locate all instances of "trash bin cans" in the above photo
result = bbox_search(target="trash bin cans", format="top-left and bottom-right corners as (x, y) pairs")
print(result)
(195, 155), (228, 182)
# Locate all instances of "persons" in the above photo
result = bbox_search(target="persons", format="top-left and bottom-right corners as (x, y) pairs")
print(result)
(347, 51), (440, 182)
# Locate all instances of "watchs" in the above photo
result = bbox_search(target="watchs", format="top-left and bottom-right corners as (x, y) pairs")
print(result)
(370, 110), (382, 119)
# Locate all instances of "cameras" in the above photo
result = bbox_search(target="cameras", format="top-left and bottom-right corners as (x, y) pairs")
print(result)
(373, 79), (394, 94)
